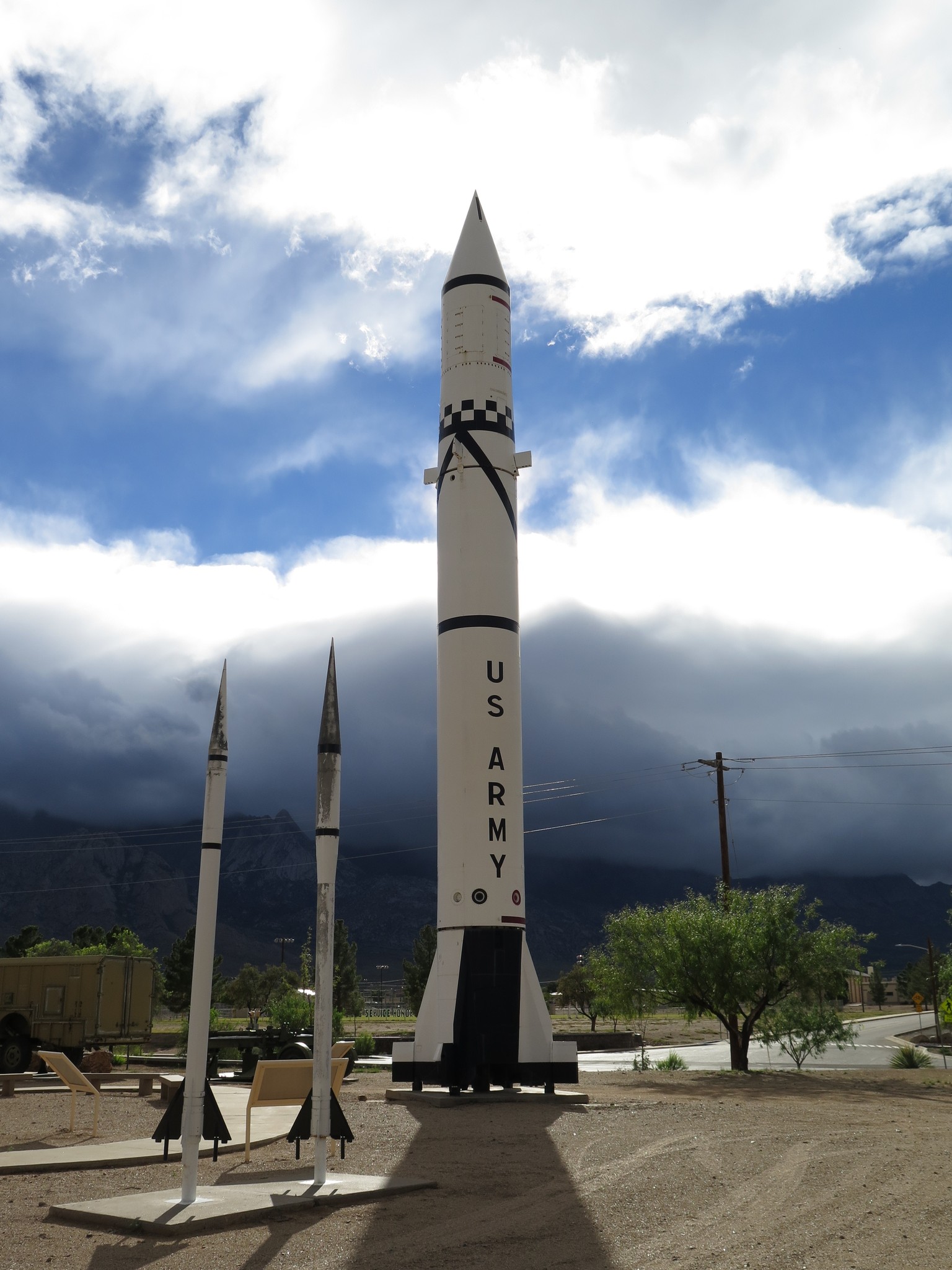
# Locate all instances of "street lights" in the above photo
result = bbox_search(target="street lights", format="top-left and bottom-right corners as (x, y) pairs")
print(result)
(895, 943), (942, 1044)
(376, 965), (389, 1017)
(274, 937), (295, 983)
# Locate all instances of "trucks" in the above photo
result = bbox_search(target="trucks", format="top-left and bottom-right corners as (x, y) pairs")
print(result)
(0, 953), (156, 1076)
